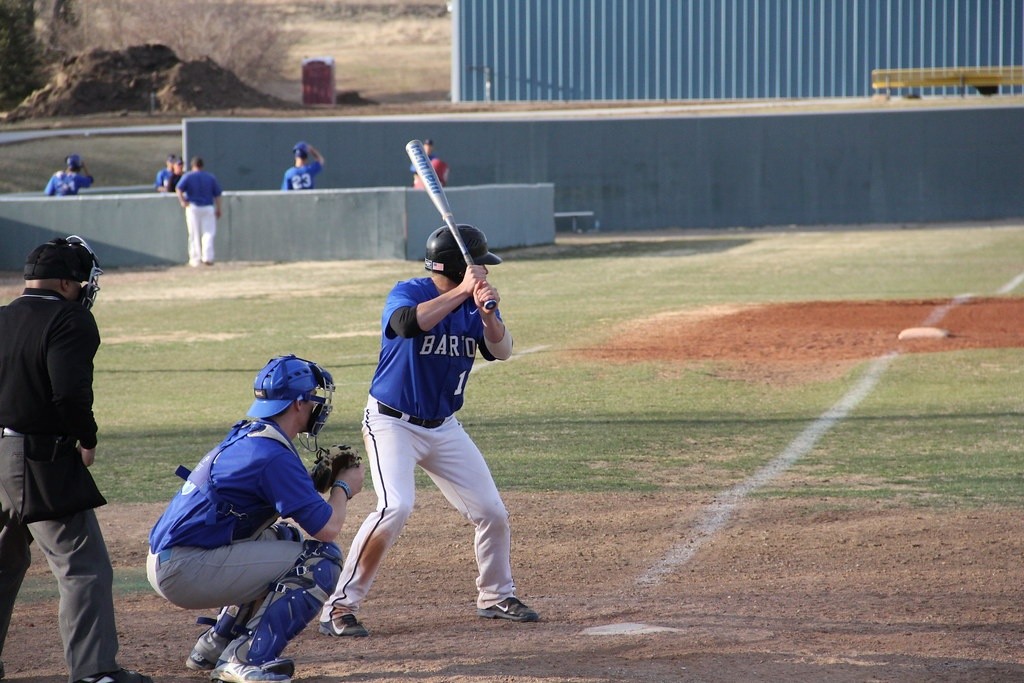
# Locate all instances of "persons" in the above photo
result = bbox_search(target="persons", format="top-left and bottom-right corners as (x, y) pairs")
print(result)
(411, 140), (439, 185)
(147, 355), (365, 683)
(155, 157), (184, 192)
(318, 224), (538, 636)
(45, 155), (94, 197)
(281, 143), (325, 190)
(176, 157), (222, 267)
(0, 235), (152, 683)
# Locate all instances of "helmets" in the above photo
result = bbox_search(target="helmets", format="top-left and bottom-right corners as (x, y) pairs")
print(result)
(292, 143), (308, 155)
(422, 224), (501, 270)
(65, 154), (81, 167)
(247, 356), (314, 417)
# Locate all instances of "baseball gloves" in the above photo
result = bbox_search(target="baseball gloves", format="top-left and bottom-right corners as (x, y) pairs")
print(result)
(309, 443), (363, 494)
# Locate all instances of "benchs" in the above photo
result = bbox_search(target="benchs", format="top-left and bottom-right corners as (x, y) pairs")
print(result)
(555, 211), (594, 232)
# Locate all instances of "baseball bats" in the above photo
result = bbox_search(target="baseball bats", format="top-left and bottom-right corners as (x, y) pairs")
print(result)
(404, 140), (497, 309)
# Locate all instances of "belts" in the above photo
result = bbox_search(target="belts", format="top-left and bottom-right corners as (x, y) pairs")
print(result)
(1, 427), (25, 438)
(379, 403), (445, 429)
(156, 548), (173, 563)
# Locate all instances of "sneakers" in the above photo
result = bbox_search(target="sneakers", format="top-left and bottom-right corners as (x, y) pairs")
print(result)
(211, 657), (289, 683)
(77, 667), (151, 683)
(477, 597), (538, 621)
(185, 648), (216, 670)
(318, 613), (368, 638)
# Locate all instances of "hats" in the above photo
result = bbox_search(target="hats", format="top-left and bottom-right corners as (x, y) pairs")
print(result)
(171, 157), (182, 164)
(23, 236), (92, 282)
(166, 154), (174, 162)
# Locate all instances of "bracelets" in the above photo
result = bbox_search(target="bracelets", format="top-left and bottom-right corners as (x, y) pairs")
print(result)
(331, 481), (352, 502)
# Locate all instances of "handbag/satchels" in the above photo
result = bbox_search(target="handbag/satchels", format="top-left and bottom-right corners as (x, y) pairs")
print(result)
(19, 448), (108, 520)
